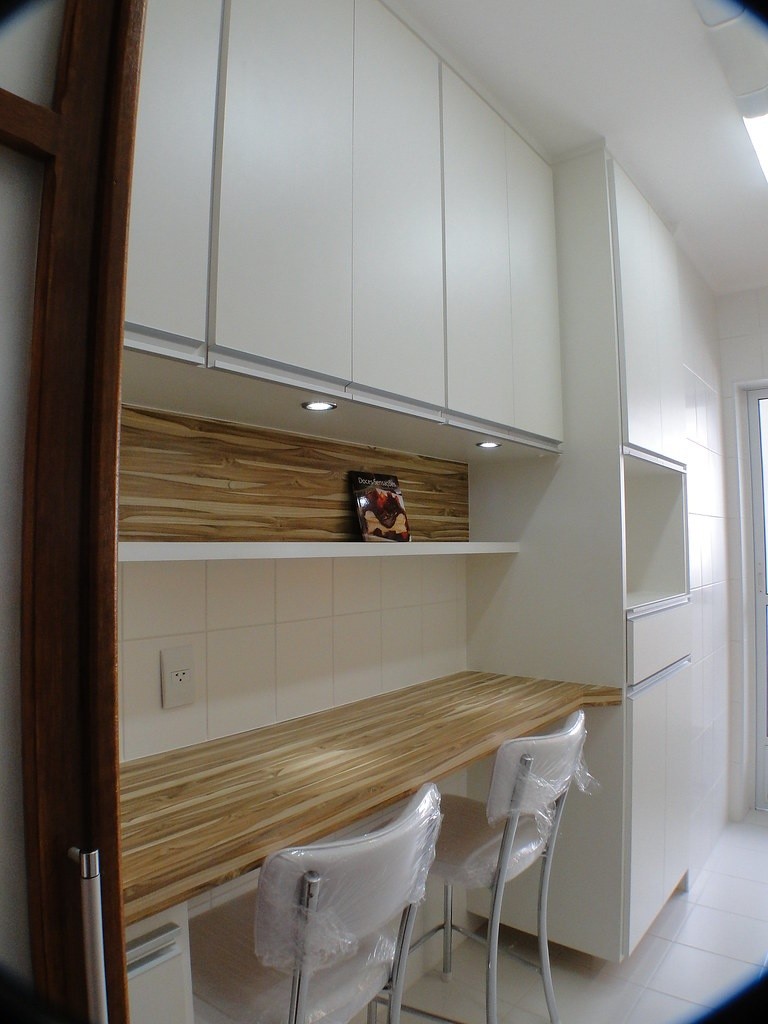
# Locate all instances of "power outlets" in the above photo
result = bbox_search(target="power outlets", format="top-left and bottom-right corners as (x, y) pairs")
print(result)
(159, 645), (195, 708)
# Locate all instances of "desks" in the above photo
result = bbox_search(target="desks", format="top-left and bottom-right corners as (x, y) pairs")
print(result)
(119, 672), (623, 1024)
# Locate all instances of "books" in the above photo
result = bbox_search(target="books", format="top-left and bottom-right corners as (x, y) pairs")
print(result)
(348, 471), (412, 542)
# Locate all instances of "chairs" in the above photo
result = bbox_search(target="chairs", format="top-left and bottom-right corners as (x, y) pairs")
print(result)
(365, 708), (589, 1024)
(252, 783), (444, 1024)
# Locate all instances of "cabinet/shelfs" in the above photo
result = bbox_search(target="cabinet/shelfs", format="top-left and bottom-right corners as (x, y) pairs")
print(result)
(124, 0), (731, 967)
